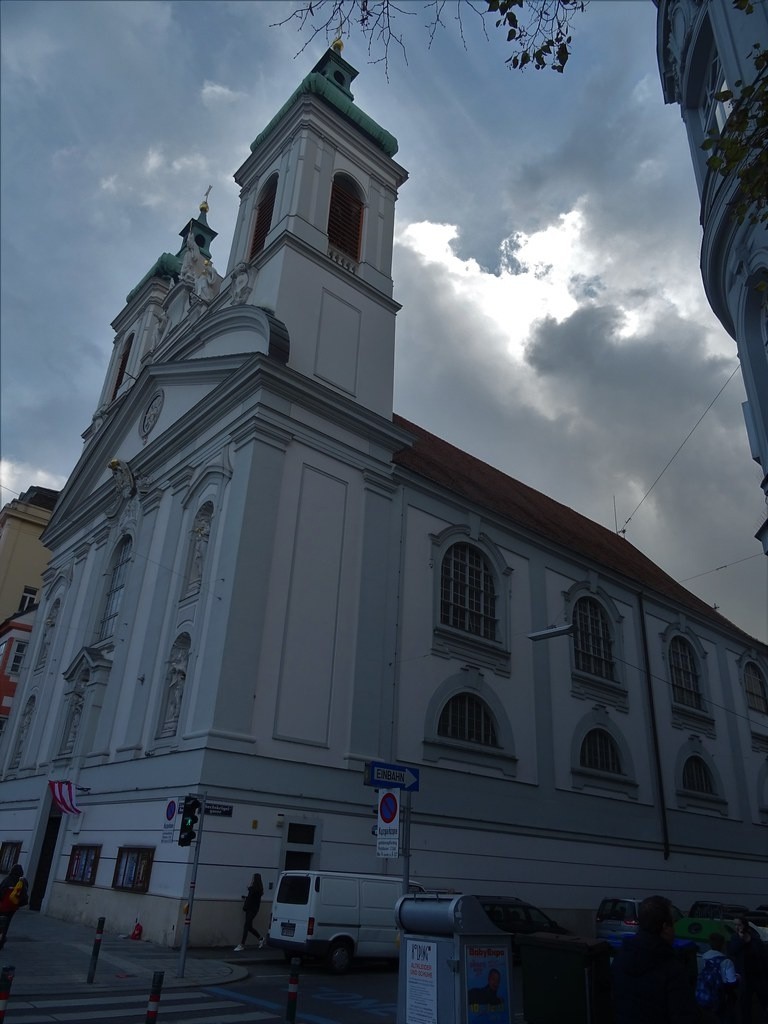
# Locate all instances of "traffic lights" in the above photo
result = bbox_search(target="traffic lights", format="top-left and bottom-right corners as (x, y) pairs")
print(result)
(177, 796), (202, 848)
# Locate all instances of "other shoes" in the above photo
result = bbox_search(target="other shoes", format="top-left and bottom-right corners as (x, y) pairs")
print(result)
(234, 945), (244, 952)
(258, 937), (266, 949)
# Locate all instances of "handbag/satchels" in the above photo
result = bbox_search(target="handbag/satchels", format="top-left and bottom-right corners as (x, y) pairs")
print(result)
(10, 877), (23, 904)
(243, 897), (247, 911)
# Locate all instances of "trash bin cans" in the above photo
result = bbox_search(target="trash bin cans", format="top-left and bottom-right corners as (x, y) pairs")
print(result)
(392, 888), (527, 1024)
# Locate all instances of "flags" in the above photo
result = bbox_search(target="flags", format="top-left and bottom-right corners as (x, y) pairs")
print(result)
(47, 780), (81, 816)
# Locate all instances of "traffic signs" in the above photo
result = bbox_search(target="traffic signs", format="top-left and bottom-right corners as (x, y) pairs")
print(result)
(363, 760), (420, 793)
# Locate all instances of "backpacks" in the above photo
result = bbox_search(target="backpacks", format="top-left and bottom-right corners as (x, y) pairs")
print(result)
(693, 956), (729, 1012)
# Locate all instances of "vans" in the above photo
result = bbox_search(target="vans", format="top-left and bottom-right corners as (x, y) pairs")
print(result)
(265, 870), (427, 976)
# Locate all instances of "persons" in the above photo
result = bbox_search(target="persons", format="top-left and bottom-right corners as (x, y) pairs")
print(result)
(696, 916), (768, 1024)
(589, 894), (715, 1023)
(233, 873), (266, 951)
(0, 864), (28, 947)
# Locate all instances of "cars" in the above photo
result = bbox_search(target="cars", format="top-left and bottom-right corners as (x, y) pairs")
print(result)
(689, 901), (749, 922)
(596, 896), (683, 952)
(476, 895), (577, 956)
